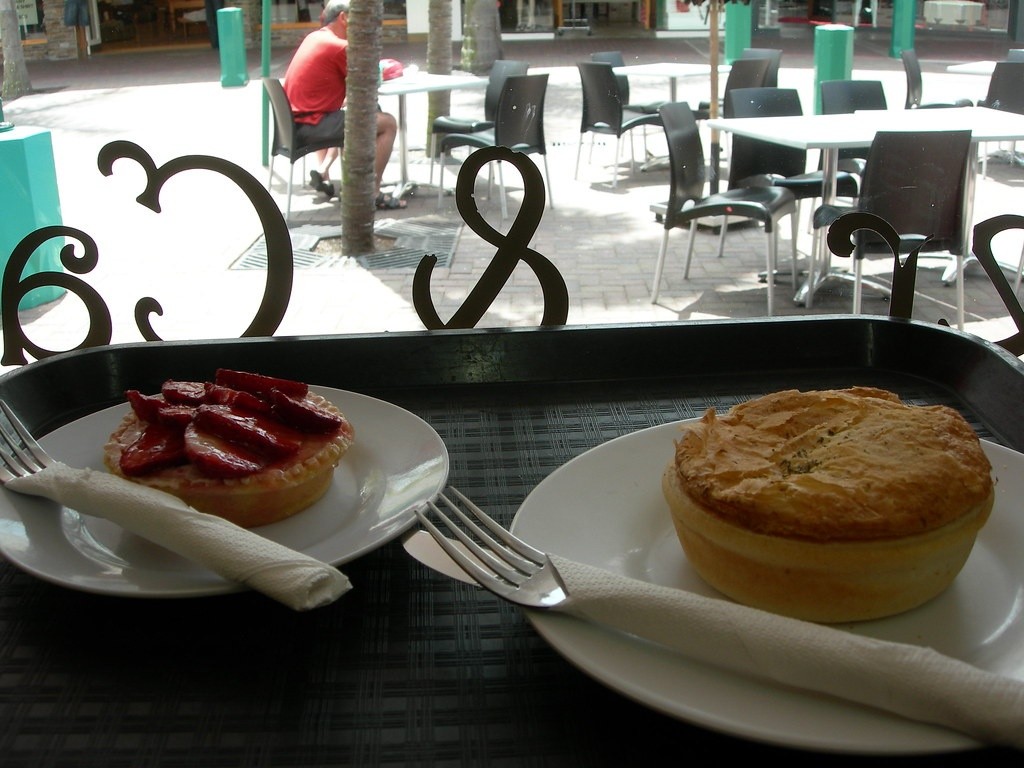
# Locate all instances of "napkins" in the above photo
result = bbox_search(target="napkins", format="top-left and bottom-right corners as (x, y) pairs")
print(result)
(554, 550), (1024, 751)
(8, 463), (353, 611)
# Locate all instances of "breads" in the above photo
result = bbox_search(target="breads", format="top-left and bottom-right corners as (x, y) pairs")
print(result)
(662, 385), (995, 624)
(106, 368), (354, 527)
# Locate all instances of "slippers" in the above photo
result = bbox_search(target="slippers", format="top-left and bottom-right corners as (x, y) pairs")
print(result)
(310, 170), (334, 196)
(375, 193), (408, 210)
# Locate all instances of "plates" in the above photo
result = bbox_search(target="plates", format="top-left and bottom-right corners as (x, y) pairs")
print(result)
(0, 387), (451, 597)
(504, 416), (1023, 750)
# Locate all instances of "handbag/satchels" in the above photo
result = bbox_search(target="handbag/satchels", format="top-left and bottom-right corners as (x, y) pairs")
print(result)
(379, 58), (403, 81)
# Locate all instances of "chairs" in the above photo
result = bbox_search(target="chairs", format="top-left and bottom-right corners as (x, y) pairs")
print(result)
(268, 48), (1024, 355)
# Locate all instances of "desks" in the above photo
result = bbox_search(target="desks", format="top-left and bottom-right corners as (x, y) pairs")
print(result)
(366, 64), (487, 198)
(524, 61), (733, 170)
(693, 105), (1021, 305)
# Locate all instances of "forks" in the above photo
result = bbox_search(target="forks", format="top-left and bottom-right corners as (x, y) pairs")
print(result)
(0, 400), (56, 481)
(411, 485), (568, 610)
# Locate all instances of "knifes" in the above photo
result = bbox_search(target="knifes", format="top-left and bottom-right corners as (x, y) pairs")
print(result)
(402, 530), (520, 587)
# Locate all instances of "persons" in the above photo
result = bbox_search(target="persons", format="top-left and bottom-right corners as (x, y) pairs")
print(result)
(282, 0), (407, 210)
